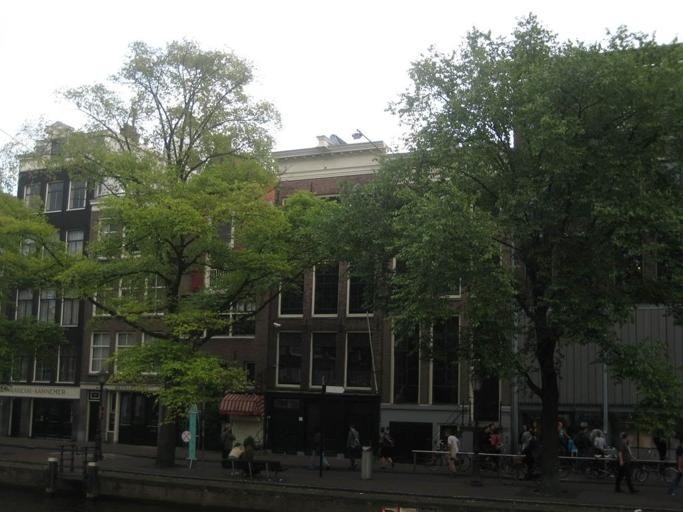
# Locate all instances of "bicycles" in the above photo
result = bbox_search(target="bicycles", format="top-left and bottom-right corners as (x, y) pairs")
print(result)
(424, 443), (680, 484)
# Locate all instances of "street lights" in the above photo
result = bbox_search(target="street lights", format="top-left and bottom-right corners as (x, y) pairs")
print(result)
(319, 372), (327, 478)
(95, 368), (111, 462)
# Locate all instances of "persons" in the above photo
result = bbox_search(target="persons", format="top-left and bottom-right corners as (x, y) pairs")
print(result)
(238, 435), (257, 463)
(346, 425), (359, 472)
(444, 429), (463, 473)
(229, 443), (242, 461)
(220, 423), (236, 470)
(480, 420), (607, 481)
(613, 433), (642, 494)
(666, 443), (683, 495)
(378, 427), (396, 469)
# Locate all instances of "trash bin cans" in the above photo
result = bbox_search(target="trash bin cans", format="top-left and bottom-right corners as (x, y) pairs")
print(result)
(360, 446), (373, 480)
